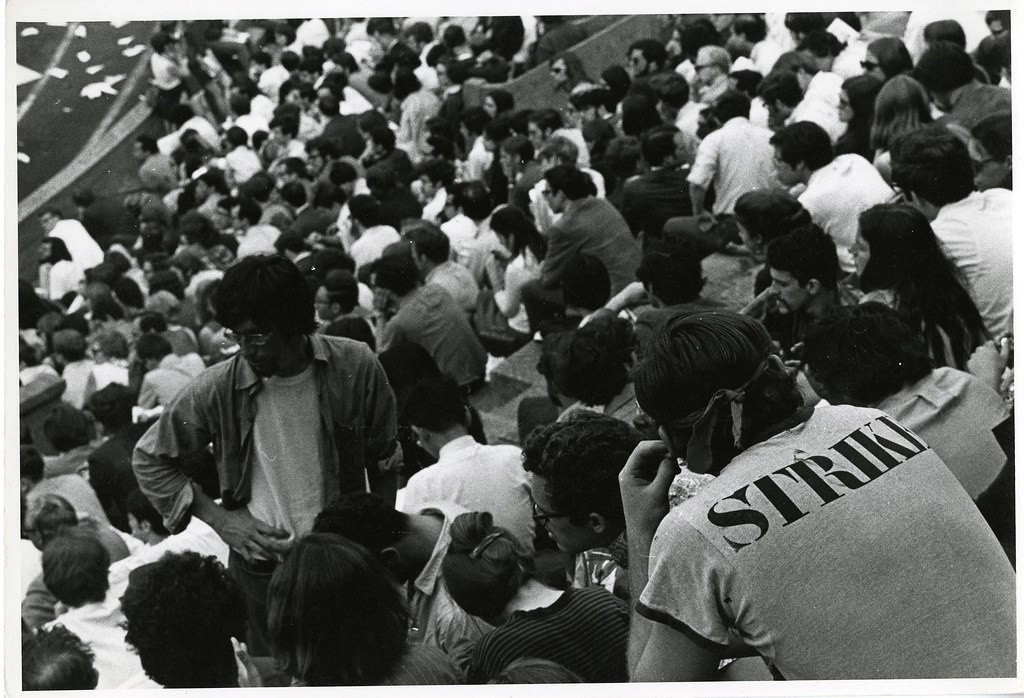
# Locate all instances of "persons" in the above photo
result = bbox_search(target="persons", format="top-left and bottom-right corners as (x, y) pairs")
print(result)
(318, 96), (366, 161)
(16, 9), (1014, 691)
(409, 227), (482, 315)
(443, 511), (628, 684)
(617, 308), (1016, 682)
(229, 199), (280, 262)
(132, 254), (402, 687)
(223, 126), (263, 191)
(540, 165), (641, 299)
(135, 335), (205, 408)
(770, 122), (897, 281)
(647, 71), (708, 137)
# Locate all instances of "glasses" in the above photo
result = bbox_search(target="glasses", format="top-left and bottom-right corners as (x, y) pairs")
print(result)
(223, 323), (290, 346)
(542, 189), (557, 197)
(625, 57), (646, 65)
(534, 503), (612, 527)
(551, 67), (565, 73)
(892, 181), (903, 194)
(971, 157), (999, 177)
(860, 59), (881, 72)
